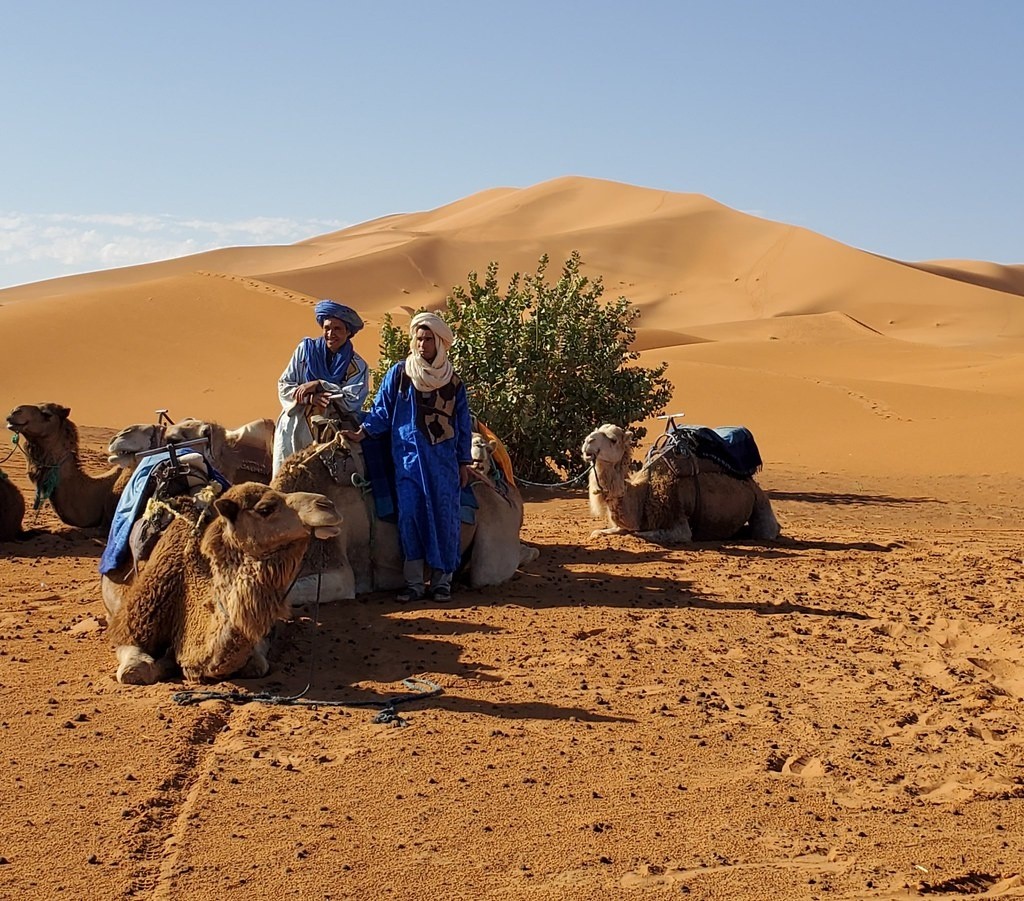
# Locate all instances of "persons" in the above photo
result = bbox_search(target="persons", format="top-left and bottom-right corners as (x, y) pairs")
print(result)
(272, 300), (369, 486)
(338, 312), (472, 602)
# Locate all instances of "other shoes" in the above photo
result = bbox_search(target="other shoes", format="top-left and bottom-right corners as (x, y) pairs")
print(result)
(396, 588), (417, 600)
(433, 586), (451, 601)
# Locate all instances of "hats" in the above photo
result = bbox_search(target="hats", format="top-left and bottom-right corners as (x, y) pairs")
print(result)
(410, 312), (452, 350)
(315, 300), (364, 338)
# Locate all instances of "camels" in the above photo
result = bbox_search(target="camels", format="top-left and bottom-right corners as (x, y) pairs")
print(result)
(107, 478), (343, 684)
(7, 398), (274, 527)
(582, 418), (781, 547)
(270, 430), (540, 588)
